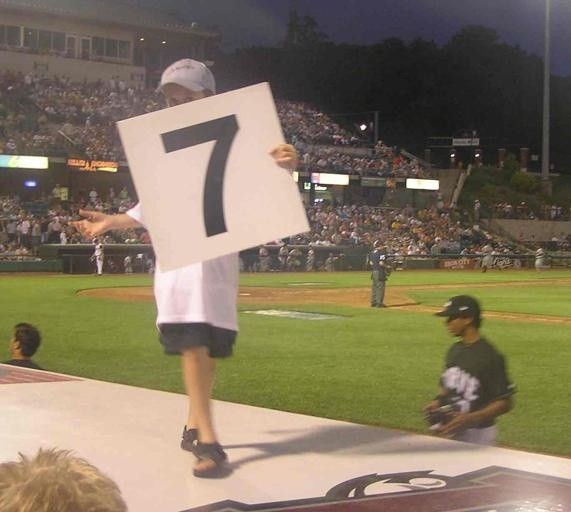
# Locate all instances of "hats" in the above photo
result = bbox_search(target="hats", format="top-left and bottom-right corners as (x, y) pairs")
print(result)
(373, 240), (385, 248)
(154, 57), (216, 95)
(434, 295), (481, 319)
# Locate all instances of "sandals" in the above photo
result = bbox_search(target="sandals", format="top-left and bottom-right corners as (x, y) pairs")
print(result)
(179, 424), (227, 478)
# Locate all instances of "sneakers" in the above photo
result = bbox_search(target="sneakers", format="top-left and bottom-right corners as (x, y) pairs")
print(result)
(371, 302), (387, 308)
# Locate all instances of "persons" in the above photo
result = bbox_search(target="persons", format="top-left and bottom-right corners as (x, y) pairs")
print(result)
(367, 238), (394, 308)
(0, 322), (45, 370)
(0, 448), (127, 512)
(66, 57), (299, 479)
(421, 294), (518, 444)
